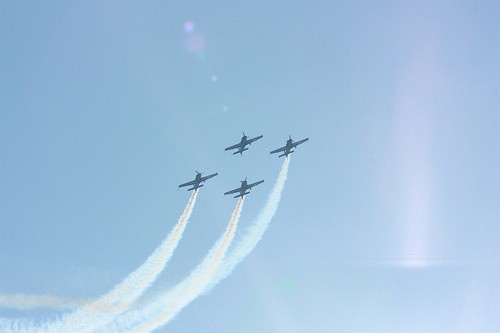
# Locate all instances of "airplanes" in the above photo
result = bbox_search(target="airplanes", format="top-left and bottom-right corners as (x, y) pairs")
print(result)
(269, 134), (310, 158)
(223, 176), (265, 200)
(178, 170), (218, 192)
(225, 132), (264, 155)
